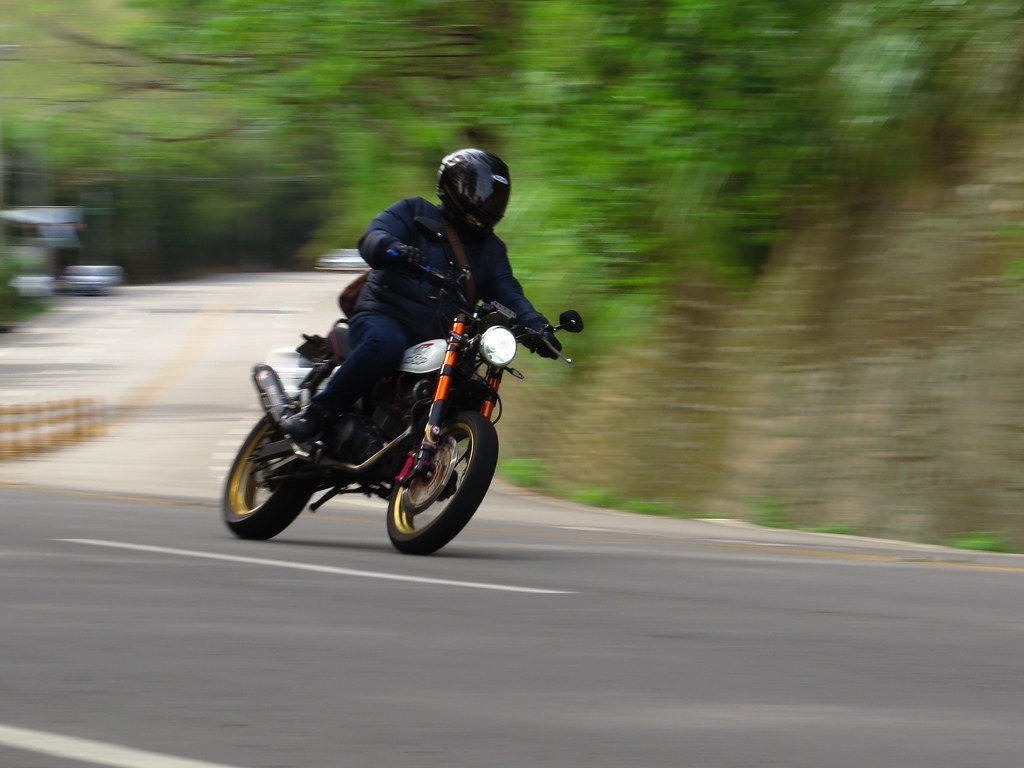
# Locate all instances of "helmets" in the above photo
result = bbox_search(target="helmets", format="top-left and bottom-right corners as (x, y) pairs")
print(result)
(435, 148), (511, 228)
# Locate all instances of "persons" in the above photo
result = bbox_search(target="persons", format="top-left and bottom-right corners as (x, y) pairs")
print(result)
(279, 149), (562, 442)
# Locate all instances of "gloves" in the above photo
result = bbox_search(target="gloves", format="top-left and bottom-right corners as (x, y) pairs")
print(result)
(538, 327), (562, 361)
(394, 242), (425, 281)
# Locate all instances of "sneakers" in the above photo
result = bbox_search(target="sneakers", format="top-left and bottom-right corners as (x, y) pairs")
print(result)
(285, 399), (326, 437)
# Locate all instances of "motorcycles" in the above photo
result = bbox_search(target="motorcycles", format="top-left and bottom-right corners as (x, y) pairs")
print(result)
(223, 215), (584, 556)
